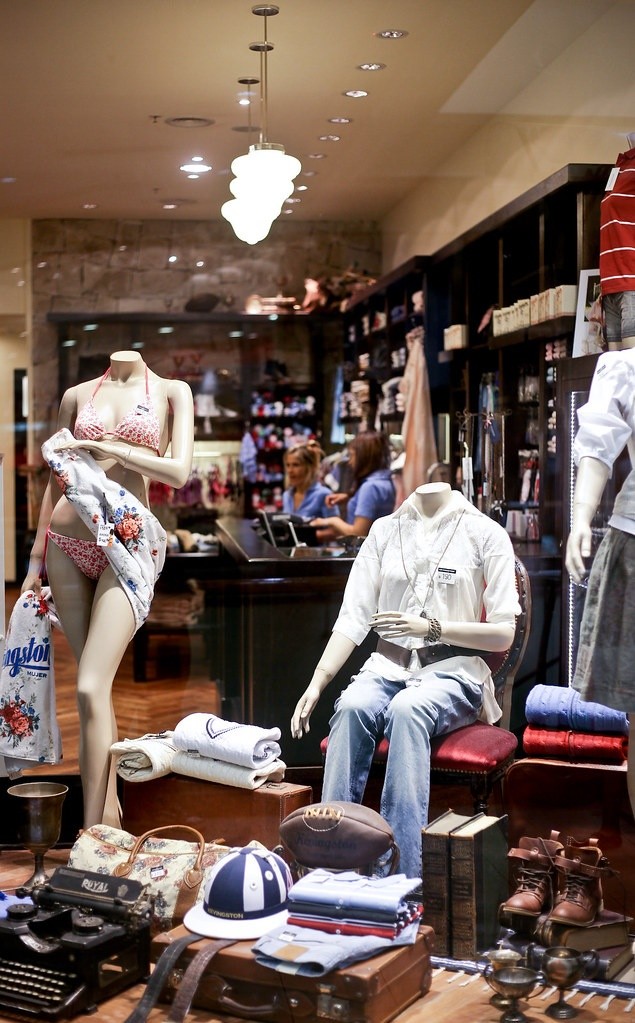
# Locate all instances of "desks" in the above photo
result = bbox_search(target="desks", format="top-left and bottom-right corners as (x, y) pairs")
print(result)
(500, 757), (628, 816)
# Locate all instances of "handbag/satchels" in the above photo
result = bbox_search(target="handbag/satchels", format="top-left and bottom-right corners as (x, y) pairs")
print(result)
(71, 824), (233, 965)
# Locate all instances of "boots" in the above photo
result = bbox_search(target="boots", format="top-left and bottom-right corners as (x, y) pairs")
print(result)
(548, 837), (621, 926)
(501, 829), (566, 918)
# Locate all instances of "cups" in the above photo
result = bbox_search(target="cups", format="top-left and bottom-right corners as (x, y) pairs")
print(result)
(521, 514), (539, 540)
(507, 510), (526, 539)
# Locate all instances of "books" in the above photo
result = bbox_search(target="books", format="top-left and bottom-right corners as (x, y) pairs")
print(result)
(421, 808), (511, 962)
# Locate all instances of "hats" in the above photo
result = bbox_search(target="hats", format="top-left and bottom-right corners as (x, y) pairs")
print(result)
(182, 844), (295, 944)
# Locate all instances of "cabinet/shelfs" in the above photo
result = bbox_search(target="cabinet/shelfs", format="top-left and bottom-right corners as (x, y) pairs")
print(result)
(45, 162), (616, 557)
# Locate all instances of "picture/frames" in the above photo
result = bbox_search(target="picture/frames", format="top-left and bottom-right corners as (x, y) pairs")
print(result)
(572, 269), (609, 358)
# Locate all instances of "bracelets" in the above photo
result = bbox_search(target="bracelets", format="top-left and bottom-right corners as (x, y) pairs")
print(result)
(316, 666), (334, 680)
(29, 553), (44, 561)
(425, 618), (442, 643)
(123, 450), (132, 466)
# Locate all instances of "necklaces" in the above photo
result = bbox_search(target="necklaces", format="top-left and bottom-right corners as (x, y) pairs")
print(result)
(398, 509), (465, 618)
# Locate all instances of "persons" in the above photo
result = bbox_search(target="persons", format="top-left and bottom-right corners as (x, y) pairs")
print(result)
(600, 132), (635, 350)
(309, 432), (396, 540)
(282, 441), (339, 546)
(565, 346), (635, 819)
(289, 481), (522, 894)
(20, 351), (194, 841)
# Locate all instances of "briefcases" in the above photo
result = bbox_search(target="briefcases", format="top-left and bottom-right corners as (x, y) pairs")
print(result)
(147, 924), (438, 1023)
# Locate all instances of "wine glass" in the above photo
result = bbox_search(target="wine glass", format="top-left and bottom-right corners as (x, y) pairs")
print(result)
(7, 783), (69, 892)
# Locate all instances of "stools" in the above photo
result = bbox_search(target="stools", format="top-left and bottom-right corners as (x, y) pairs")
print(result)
(319, 721), (520, 816)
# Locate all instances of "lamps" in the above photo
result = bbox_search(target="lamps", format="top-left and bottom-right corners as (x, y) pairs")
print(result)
(222, 5), (300, 245)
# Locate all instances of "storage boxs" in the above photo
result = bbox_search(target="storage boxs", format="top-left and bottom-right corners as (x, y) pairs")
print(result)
(492, 284), (578, 335)
